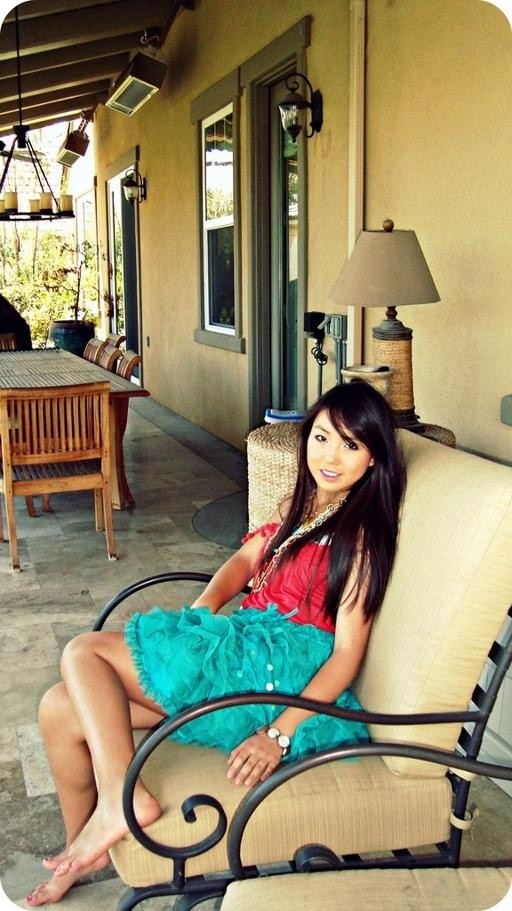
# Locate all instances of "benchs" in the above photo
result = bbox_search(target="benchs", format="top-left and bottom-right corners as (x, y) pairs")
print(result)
(218, 744), (512, 911)
(91, 426), (511, 909)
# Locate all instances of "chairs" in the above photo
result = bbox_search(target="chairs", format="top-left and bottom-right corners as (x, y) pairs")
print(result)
(84, 337), (106, 363)
(1, 379), (120, 574)
(97, 342), (119, 373)
(105, 332), (127, 349)
(45, 348), (142, 516)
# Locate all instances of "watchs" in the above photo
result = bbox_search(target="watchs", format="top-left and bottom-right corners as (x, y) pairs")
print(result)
(260, 726), (291, 758)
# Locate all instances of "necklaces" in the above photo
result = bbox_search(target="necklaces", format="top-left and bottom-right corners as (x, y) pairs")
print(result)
(302, 489), (337, 520)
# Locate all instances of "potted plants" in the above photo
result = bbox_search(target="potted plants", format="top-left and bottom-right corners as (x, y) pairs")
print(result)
(43, 238), (100, 358)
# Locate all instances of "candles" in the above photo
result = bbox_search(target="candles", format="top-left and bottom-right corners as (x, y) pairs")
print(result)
(0, 190), (74, 213)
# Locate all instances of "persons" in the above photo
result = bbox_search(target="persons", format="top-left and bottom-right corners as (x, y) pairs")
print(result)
(18, 378), (411, 906)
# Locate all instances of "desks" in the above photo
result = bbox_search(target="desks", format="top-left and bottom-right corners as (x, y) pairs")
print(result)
(7, 348), (151, 512)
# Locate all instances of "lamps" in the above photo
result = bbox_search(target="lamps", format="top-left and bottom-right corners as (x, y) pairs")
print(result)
(103, 50), (168, 118)
(56, 104), (100, 169)
(278, 70), (317, 144)
(327, 215), (442, 430)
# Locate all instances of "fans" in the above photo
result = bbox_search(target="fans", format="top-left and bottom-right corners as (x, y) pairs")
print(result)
(0, 140), (45, 163)
